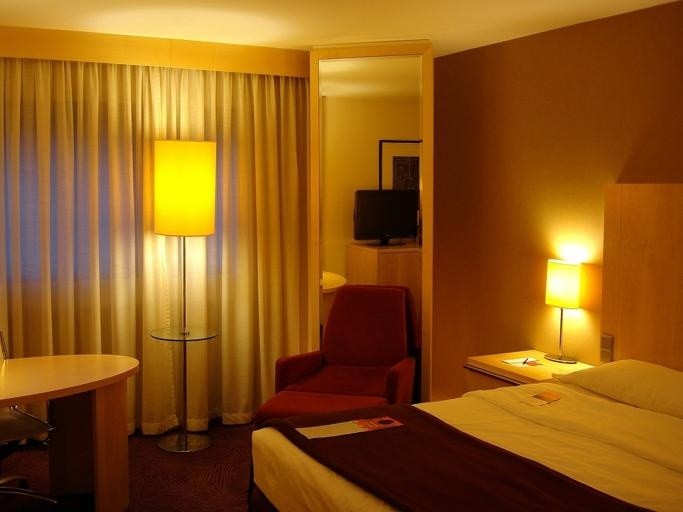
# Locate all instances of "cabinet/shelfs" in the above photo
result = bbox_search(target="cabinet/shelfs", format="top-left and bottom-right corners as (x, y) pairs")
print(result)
(345, 242), (420, 334)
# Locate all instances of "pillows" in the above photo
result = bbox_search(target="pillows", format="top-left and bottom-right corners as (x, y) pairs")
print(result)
(547, 358), (683, 420)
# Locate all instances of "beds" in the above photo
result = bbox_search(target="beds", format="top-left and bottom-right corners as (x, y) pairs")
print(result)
(245, 184), (683, 512)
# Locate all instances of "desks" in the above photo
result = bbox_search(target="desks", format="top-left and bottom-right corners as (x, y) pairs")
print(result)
(0, 353), (140, 511)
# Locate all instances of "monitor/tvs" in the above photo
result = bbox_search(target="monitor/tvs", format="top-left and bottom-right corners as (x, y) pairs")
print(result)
(352, 188), (420, 247)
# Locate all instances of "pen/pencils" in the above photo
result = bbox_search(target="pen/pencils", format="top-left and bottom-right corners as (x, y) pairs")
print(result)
(523, 359), (528, 364)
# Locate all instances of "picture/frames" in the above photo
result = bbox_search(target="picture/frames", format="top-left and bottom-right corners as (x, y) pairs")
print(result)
(378, 139), (421, 192)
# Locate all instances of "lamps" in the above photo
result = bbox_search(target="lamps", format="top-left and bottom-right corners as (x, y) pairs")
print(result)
(145, 137), (223, 454)
(544, 258), (583, 365)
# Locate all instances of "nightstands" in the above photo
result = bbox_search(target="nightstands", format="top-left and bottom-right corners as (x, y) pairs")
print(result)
(462, 349), (596, 395)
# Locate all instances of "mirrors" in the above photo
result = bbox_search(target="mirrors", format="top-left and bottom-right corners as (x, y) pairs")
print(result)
(308, 42), (436, 403)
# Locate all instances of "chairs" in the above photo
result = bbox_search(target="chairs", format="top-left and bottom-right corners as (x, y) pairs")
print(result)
(0, 330), (64, 510)
(255, 285), (418, 430)
